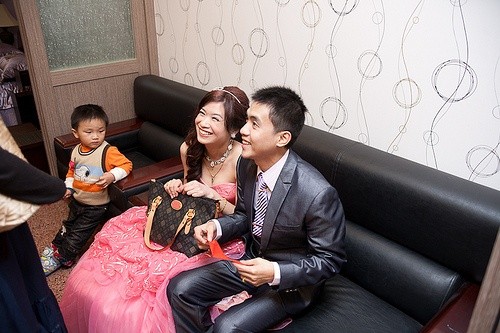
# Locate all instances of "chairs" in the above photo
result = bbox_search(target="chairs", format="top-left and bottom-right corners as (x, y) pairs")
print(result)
(53, 73), (209, 223)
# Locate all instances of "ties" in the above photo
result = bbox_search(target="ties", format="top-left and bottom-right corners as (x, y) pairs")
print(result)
(252, 176), (268, 237)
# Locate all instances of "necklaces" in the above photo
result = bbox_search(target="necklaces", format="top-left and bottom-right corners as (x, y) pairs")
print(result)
(203, 139), (234, 183)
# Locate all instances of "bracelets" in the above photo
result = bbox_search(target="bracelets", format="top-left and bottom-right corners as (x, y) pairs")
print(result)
(219, 198), (228, 213)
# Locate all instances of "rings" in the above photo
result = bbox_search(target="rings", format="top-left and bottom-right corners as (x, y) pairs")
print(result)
(241, 276), (246, 283)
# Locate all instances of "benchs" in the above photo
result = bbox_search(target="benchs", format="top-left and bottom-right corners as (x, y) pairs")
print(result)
(126, 124), (500, 333)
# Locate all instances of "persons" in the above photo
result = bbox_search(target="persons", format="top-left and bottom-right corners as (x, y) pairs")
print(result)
(68, 86), (347, 333)
(0, 114), (66, 333)
(40, 104), (133, 277)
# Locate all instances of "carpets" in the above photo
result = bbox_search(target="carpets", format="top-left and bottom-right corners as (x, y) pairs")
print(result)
(21, 141), (51, 176)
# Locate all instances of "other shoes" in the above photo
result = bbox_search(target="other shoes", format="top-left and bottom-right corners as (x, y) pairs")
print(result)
(40, 246), (64, 277)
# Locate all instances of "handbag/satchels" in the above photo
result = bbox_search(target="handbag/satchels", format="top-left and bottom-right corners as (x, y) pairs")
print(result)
(143, 178), (220, 259)
